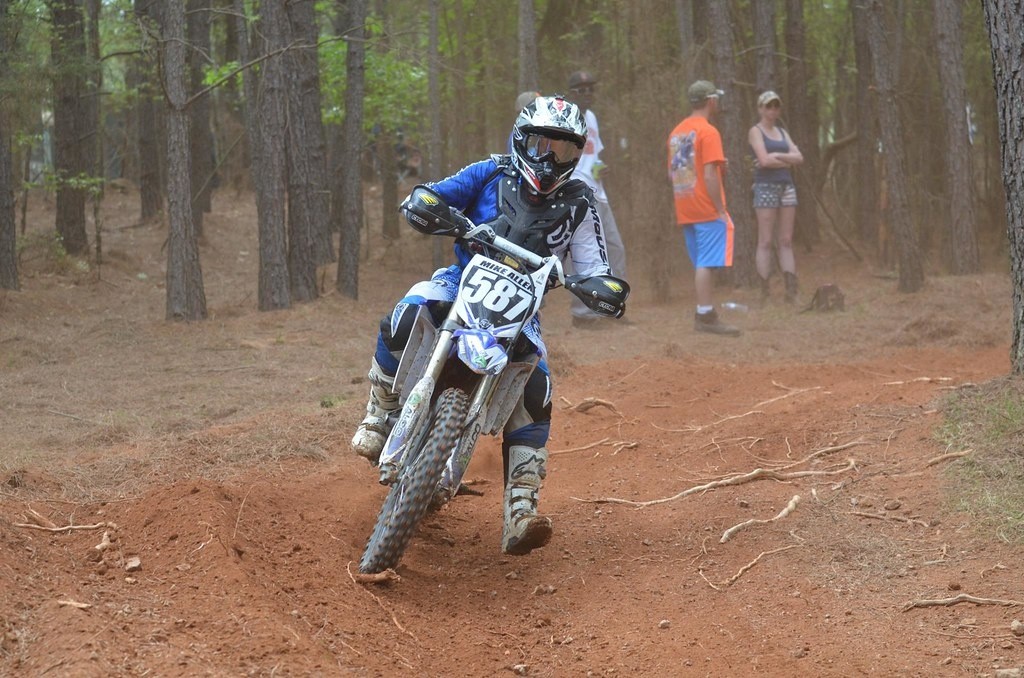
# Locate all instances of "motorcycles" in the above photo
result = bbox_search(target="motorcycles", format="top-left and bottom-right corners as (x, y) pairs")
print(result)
(359, 197), (625, 576)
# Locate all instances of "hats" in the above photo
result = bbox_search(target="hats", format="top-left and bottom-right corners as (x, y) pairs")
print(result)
(758, 92), (782, 107)
(570, 72), (596, 88)
(688, 81), (725, 101)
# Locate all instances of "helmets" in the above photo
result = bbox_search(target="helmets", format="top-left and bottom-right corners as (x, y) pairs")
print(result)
(510, 93), (588, 196)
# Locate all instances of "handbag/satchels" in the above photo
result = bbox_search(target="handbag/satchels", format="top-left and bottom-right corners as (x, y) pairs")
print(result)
(818, 283), (844, 313)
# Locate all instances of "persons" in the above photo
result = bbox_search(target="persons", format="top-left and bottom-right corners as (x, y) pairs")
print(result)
(747, 90), (810, 313)
(507, 67), (634, 332)
(353, 93), (626, 555)
(667, 79), (742, 337)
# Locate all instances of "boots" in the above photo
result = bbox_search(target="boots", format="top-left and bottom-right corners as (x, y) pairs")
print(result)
(785, 269), (804, 308)
(759, 270), (772, 298)
(350, 355), (402, 459)
(501, 440), (554, 554)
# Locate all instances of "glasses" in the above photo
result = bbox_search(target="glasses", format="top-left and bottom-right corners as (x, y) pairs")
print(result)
(524, 132), (584, 167)
(571, 87), (593, 93)
(763, 103), (780, 109)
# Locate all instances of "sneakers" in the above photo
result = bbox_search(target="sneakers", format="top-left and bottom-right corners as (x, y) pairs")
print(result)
(696, 313), (739, 336)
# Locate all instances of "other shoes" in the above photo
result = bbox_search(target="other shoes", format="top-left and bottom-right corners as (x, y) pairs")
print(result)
(573, 316), (609, 332)
